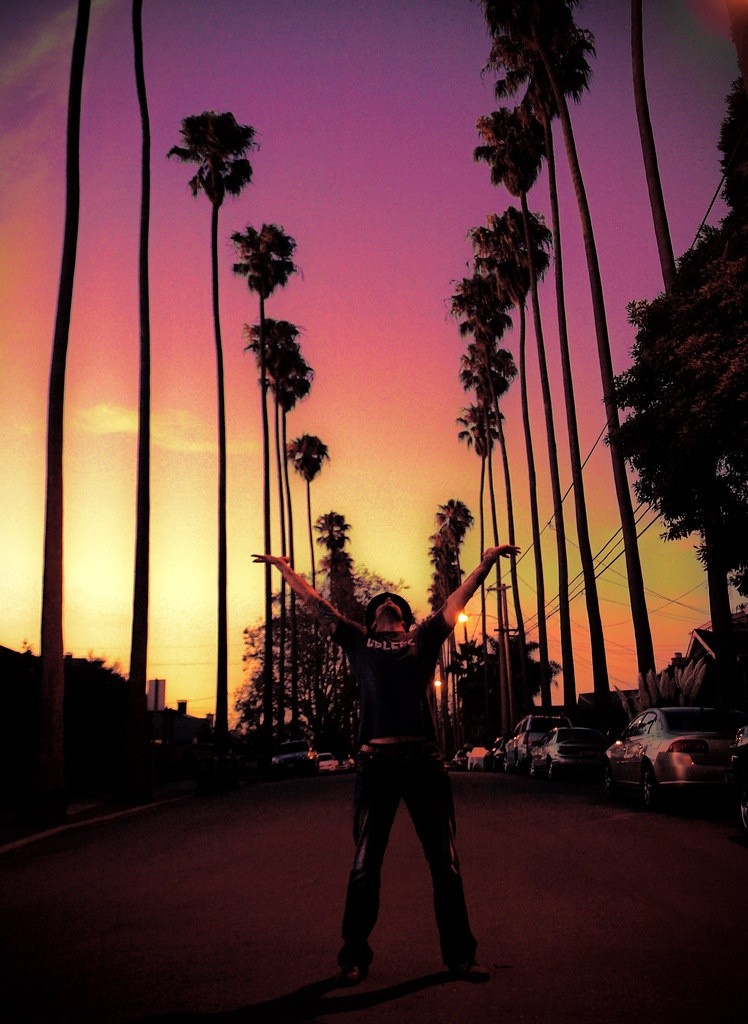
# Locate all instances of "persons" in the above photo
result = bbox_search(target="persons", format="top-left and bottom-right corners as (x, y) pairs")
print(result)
(251, 545), (522, 987)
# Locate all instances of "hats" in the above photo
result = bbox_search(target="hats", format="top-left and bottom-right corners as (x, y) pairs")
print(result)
(366, 592), (412, 630)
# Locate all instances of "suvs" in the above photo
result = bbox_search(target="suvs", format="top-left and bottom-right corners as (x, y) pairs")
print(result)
(504, 714), (573, 777)
(271, 740), (316, 777)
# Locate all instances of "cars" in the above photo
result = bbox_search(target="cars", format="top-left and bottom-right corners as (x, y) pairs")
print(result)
(317, 752), (337, 773)
(450, 735), (509, 772)
(523, 726), (610, 785)
(603, 705), (746, 818)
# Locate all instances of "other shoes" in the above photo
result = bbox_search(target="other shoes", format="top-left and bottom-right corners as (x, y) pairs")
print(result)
(328, 964), (367, 985)
(448, 961), (490, 983)
